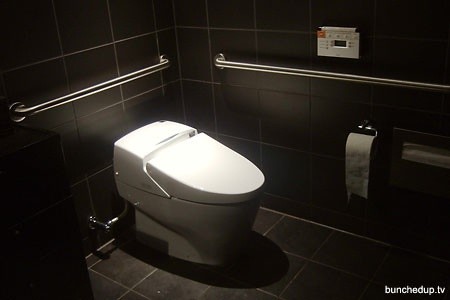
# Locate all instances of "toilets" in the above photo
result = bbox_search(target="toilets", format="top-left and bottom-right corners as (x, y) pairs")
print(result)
(113, 119), (265, 265)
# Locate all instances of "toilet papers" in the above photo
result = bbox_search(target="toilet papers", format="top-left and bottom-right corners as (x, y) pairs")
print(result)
(345, 132), (376, 202)
